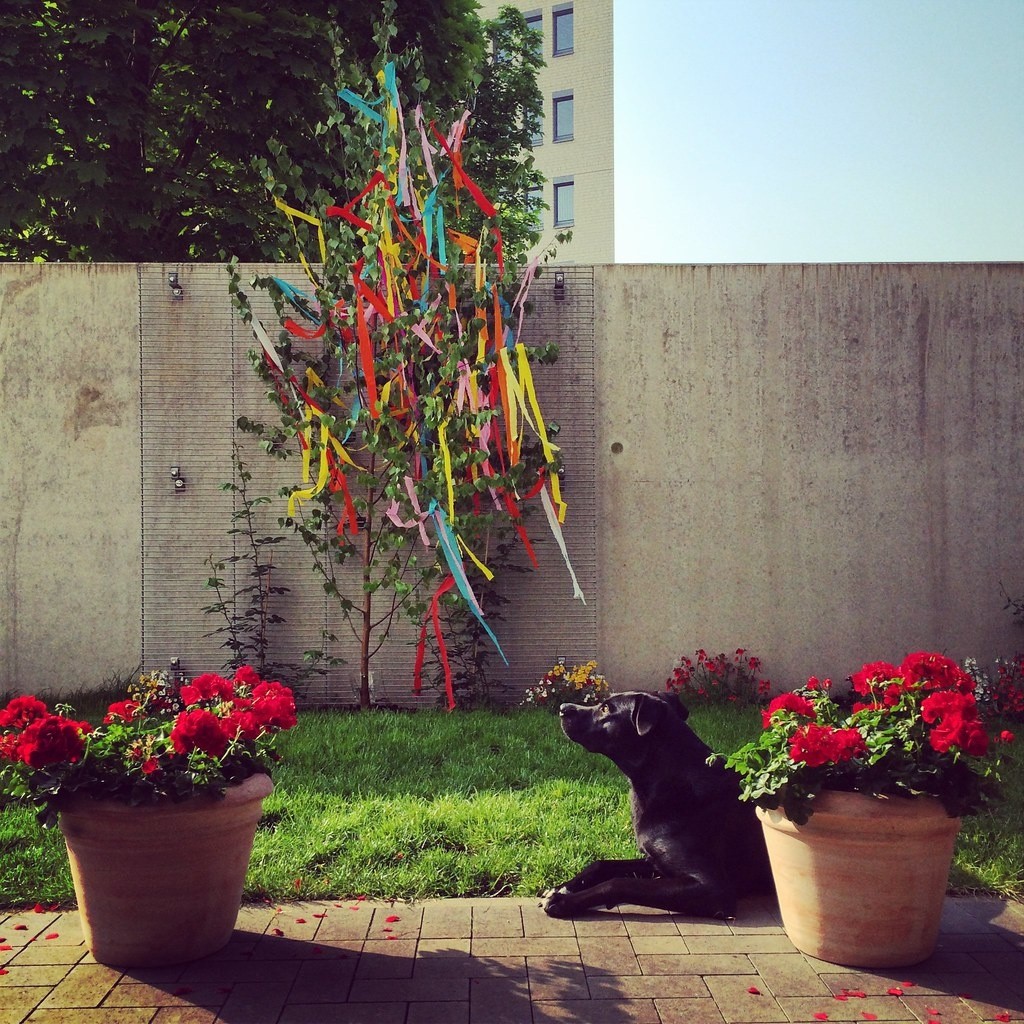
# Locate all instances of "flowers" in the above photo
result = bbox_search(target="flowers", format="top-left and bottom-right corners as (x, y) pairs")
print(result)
(705, 652), (1019, 827)
(0, 664), (297, 831)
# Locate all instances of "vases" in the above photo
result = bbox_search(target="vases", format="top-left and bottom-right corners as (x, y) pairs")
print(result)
(754, 786), (962, 966)
(57, 772), (274, 967)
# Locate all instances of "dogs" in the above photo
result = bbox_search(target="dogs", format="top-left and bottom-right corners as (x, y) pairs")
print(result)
(542, 690), (780, 923)
